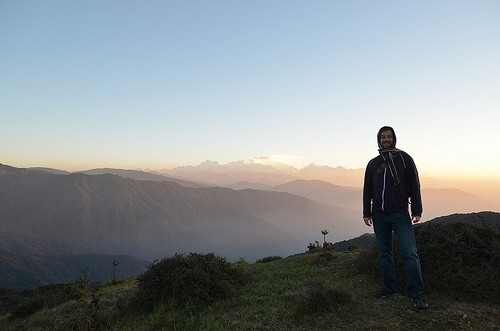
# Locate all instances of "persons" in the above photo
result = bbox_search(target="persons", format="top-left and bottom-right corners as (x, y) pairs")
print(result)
(363, 126), (428, 309)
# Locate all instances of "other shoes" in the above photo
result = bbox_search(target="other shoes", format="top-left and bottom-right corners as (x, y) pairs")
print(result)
(374, 289), (398, 299)
(411, 295), (428, 309)
